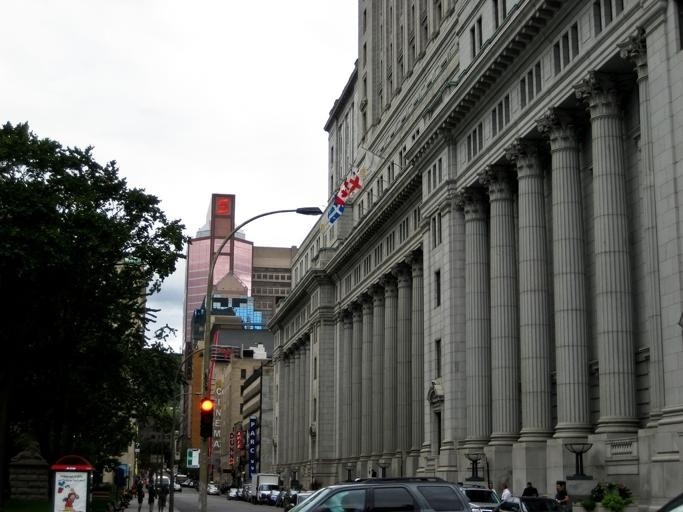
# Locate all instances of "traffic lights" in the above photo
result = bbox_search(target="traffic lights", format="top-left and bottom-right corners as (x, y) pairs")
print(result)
(199, 398), (215, 437)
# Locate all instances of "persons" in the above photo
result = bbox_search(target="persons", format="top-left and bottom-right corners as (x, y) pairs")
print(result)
(63, 493), (76, 512)
(555, 483), (569, 505)
(138, 484), (169, 512)
(501, 483), (513, 501)
(522, 482), (539, 496)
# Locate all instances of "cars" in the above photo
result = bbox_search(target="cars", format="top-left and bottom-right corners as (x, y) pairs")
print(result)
(146, 469), (220, 496)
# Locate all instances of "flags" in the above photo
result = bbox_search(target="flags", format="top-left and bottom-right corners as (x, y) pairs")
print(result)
(328, 151), (383, 225)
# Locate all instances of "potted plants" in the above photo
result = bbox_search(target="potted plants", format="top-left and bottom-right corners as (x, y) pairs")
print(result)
(572, 481), (638, 511)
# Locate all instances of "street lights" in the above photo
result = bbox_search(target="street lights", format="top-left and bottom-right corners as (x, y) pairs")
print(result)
(198, 207), (324, 512)
